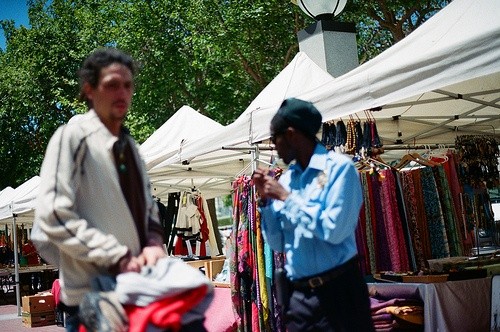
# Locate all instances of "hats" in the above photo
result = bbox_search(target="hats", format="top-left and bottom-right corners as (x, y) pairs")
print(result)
(277, 99), (322, 134)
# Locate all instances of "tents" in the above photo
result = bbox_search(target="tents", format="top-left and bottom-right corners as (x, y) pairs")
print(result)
(0, 0), (500, 317)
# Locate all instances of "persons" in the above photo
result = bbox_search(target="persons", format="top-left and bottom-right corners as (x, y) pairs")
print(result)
(250, 97), (378, 332)
(31, 46), (163, 332)
(0, 238), (65, 328)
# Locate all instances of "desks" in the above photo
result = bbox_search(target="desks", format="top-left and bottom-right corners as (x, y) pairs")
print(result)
(367, 277), (492, 332)
(0, 266), (57, 275)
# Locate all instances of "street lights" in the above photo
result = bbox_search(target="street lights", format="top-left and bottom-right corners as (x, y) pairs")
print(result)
(296, 0), (360, 78)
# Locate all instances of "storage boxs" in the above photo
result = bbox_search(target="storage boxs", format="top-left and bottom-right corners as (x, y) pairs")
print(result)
(21, 312), (56, 328)
(21, 294), (55, 313)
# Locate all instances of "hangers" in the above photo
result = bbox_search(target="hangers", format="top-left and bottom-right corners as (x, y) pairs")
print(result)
(455, 114), (496, 138)
(233, 109), (449, 181)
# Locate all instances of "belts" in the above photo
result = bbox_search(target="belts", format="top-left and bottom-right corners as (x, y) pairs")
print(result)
(290, 274), (334, 289)
